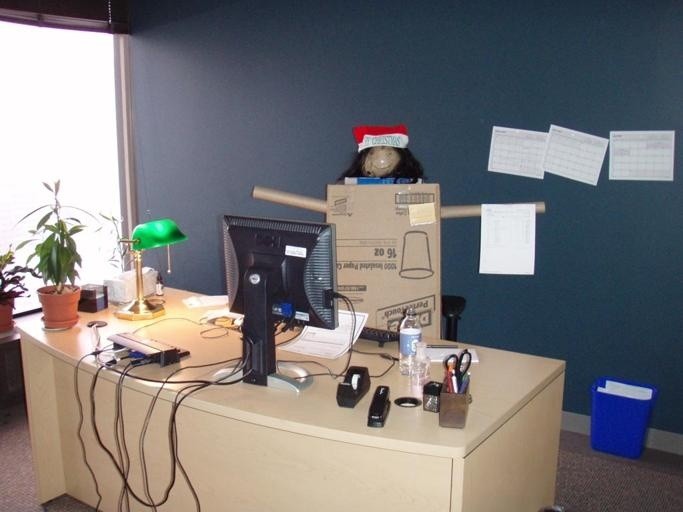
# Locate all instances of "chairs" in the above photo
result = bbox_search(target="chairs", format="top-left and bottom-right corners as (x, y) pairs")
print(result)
(439, 296), (465, 342)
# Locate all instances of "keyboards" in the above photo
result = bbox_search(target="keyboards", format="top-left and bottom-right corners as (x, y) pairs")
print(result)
(359, 326), (399, 348)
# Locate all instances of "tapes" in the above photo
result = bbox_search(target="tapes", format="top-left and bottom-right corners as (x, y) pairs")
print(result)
(351, 374), (360, 390)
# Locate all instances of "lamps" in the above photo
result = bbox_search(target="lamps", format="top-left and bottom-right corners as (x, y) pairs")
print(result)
(109, 218), (190, 321)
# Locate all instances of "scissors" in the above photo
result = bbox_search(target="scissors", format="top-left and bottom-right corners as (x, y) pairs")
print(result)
(445, 349), (472, 383)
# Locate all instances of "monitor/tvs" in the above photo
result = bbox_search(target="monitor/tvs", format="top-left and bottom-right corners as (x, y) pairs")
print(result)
(212, 215), (339, 395)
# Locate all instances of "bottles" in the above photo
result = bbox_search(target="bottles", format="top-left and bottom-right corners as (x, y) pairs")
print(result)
(399, 306), (422, 375)
(408, 340), (433, 395)
(156, 273), (163, 296)
(423, 381), (443, 412)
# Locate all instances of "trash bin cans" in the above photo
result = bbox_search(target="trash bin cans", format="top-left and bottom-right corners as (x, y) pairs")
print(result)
(588, 375), (659, 459)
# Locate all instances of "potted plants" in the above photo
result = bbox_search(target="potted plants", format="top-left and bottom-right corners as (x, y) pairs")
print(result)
(0, 239), (45, 335)
(13, 178), (99, 331)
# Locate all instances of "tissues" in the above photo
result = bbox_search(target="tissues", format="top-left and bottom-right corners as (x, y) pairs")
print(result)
(103, 267), (158, 304)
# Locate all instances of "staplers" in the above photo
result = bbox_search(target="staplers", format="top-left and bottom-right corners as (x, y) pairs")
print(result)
(367, 385), (390, 428)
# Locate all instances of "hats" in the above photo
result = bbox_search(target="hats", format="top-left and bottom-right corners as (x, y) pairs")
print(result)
(352, 124), (408, 152)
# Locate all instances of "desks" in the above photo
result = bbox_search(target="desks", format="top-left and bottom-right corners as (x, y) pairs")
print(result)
(9, 286), (567, 511)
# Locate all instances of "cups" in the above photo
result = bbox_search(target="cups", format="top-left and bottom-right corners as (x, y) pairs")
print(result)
(438, 389), (469, 430)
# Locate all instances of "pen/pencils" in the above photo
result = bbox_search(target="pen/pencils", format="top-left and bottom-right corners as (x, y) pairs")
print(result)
(446, 369), (471, 394)
